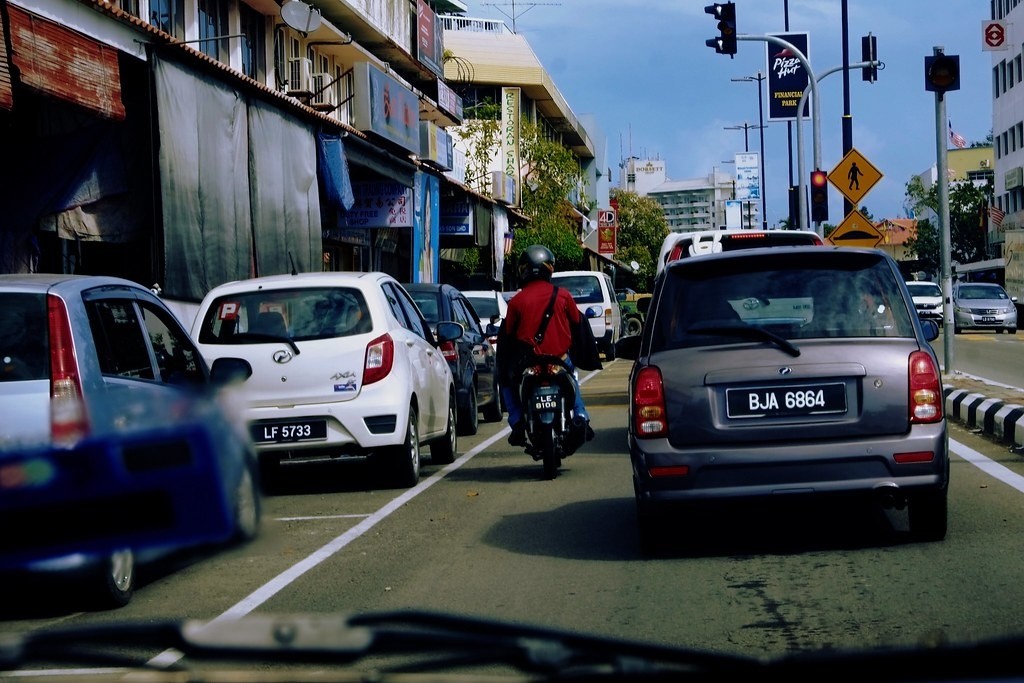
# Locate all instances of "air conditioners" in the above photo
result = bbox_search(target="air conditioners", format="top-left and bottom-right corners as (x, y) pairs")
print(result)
(309, 72), (337, 111)
(287, 56), (312, 96)
(1004, 166), (1023, 191)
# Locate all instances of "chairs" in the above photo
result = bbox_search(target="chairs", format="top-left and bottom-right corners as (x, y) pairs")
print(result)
(247, 308), (287, 337)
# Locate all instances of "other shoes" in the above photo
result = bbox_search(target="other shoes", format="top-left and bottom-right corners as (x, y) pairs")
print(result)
(507, 422), (525, 445)
(586, 426), (595, 440)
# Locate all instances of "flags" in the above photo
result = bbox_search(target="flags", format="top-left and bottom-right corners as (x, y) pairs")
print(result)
(991, 206), (1005, 227)
(949, 128), (967, 149)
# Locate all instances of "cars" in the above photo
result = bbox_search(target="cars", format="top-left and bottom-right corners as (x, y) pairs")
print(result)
(904, 281), (943, 327)
(188, 271), (464, 487)
(462, 290), (509, 346)
(0, 273), (261, 609)
(953, 282), (1018, 334)
(401, 283), (503, 436)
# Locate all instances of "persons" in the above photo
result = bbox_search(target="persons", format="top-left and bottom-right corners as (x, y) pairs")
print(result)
(499, 243), (596, 446)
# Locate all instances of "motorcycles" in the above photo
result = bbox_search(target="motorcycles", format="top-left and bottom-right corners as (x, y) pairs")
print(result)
(487, 305), (603, 478)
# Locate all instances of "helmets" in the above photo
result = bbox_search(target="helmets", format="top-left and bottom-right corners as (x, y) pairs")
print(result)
(518, 244), (555, 280)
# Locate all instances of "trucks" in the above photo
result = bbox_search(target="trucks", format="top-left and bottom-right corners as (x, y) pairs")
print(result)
(619, 300), (648, 337)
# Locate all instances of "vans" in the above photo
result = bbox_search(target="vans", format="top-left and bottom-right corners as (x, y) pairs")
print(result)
(655, 229), (825, 332)
(550, 271), (623, 362)
(616, 244), (949, 541)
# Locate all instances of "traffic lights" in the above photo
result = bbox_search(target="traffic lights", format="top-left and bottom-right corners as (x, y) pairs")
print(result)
(705, 3), (737, 54)
(811, 171), (828, 221)
(925, 54), (961, 91)
